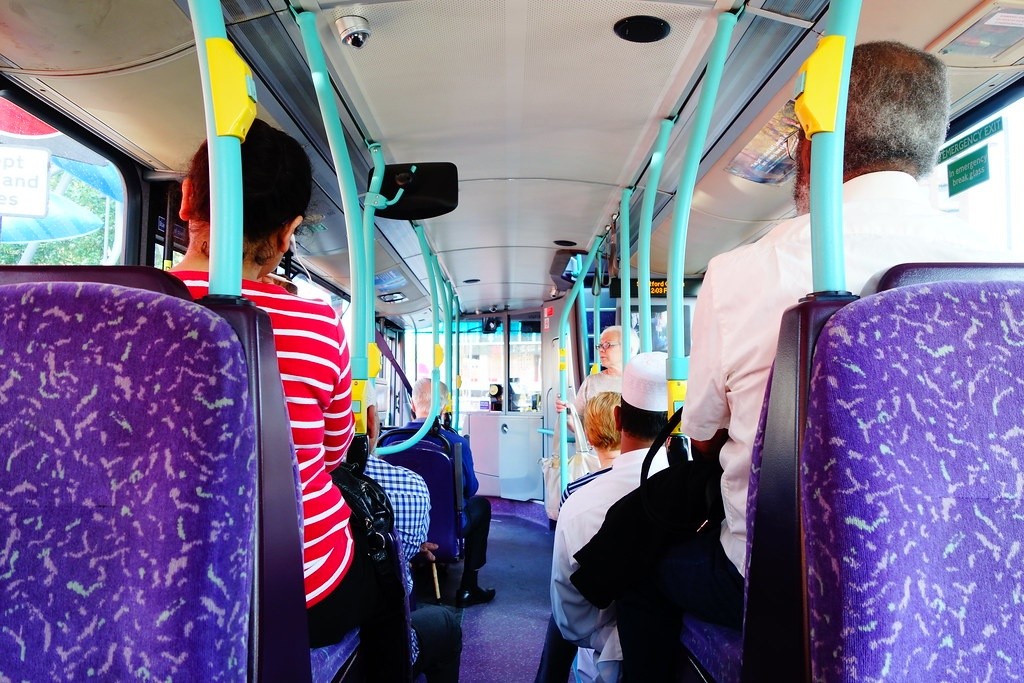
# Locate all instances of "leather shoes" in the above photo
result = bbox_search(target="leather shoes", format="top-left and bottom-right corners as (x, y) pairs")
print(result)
(457, 586), (496, 608)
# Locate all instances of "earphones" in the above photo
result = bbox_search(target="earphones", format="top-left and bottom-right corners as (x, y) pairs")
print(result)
(290, 233), (297, 253)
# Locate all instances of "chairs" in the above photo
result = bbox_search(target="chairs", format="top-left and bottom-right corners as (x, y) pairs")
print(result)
(561, 247), (1024, 683)
(0, 263), (492, 683)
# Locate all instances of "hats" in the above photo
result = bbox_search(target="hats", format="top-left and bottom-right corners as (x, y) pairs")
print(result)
(621, 351), (669, 411)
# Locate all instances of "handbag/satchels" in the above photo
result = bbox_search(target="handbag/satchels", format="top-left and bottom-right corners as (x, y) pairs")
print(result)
(328, 434), (394, 563)
(541, 401), (602, 521)
(570, 407), (726, 611)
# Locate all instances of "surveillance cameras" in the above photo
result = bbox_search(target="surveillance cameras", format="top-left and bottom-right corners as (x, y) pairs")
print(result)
(489, 306), (497, 312)
(335, 16), (372, 48)
(550, 289), (560, 298)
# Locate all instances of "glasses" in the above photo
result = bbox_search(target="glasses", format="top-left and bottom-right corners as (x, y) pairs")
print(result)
(595, 342), (622, 349)
(784, 128), (802, 161)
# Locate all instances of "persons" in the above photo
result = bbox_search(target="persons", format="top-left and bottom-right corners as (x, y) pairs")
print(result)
(405, 379), (497, 607)
(362, 382), (462, 683)
(537, 326), (693, 683)
(165, 117), (412, 683)
(614, 39), (998, 683)
